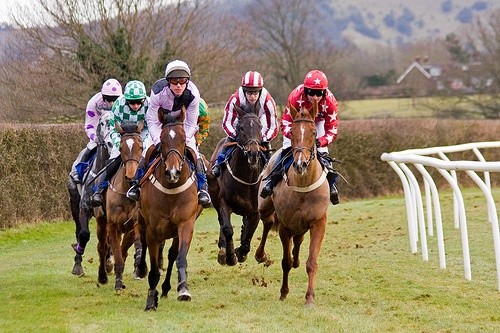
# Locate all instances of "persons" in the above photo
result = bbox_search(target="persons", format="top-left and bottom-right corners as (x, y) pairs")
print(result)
(210, 70), (280, 178)
(260, 70), (340, 206)
(93, 80), (150, 204)
(128, 59), (210, 207)
(69, 79), (122, 184)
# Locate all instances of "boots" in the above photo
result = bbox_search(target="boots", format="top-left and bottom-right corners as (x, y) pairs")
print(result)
(69, 148), (94, 184)
(93, 170), (108, 204)
(128, 159), (145, 200)
(330, 185), (339, 205)
(197, 158), (210, 209)
(260, 152), (284, 199)
(211, 138), (235, 178)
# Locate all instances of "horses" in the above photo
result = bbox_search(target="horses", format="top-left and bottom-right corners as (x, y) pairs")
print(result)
(259, 99), (330, 306)
(206, 98), (273, 266)
(68, 118), (210, 313)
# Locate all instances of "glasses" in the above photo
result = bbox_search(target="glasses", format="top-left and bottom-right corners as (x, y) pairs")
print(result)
(102, 95), (119, 103)
(305, 88), (325, 97)
(127, 100), (144, 105)
(168, 77), (189, 85)
(245, 90), (259, 95)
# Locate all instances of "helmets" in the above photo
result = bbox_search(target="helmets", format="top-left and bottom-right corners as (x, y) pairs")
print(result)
(124, 80), (146, 100)
(241, 70), (264, 91)
(304, 70), (328, 94)
(165, 60), (190, 78)
(101, 78), (122, 96)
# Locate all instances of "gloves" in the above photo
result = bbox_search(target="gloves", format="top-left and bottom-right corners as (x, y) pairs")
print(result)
(316, 139), (320, 148)
